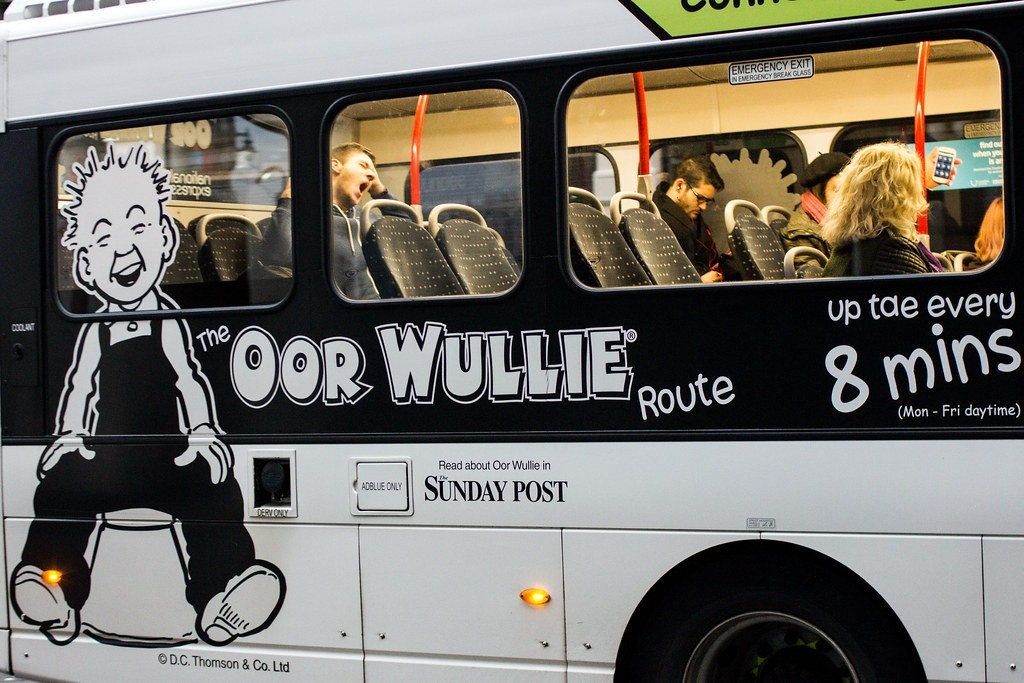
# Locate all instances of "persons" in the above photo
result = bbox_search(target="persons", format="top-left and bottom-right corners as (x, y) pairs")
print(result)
(250, 144), (413, 305)
(781, 142), (943, 279)
(967, 195), (1005, 269)
(650, 157), (744, 284)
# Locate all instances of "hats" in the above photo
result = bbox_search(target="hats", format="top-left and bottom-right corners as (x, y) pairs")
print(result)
(799, 152), (850, 189)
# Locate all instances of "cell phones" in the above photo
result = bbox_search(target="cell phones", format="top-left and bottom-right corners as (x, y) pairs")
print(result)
(933, 146), (957, 184)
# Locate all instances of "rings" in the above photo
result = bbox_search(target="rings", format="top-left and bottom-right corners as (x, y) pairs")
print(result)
(718, 273), (721, 277)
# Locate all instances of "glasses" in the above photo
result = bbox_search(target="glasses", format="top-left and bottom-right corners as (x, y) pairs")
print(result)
(686, 181), (715, 206)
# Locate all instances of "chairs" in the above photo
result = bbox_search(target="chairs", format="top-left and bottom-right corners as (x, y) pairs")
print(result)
(427, 203), (522, 300)
(928, 249), (985, 275)
(762, 204), (798, 252)
(608, 192), (703, 286)
(723, 199), (792, 279)
(193, 211), (266, 282)
(782, 245), (832, 278)
(256, 216), (274, 241)
(160, 212), (204, 283)
(357, 197), (466, 304)
(565, 184), (654, 290)
(56, 209), (81, 290)
